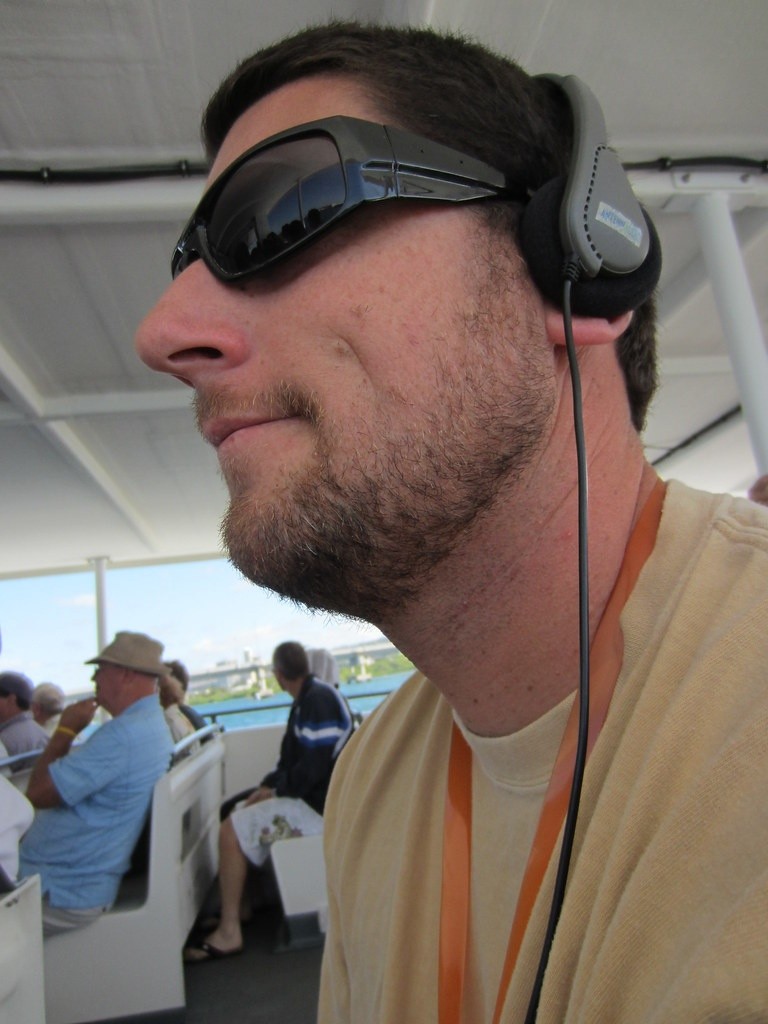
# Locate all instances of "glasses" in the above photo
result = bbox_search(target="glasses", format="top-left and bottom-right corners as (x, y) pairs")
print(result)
(170, 113), (510, 281)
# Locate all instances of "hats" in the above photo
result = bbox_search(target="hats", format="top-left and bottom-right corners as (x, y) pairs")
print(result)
(31, 684), (65, 710)
(1, 671), (35, 701)
(83, 631), (166, 677)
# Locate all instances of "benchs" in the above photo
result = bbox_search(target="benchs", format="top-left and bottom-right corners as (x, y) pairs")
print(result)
(0, 711), (367, 1024)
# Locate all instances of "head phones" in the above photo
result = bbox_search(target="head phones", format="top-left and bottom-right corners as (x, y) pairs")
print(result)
(518, 70), (664, 321)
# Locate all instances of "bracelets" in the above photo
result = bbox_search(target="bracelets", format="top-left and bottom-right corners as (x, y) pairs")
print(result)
(56, 726), (80, 739)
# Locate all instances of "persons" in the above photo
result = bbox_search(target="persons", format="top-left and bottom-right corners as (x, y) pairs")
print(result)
(218, 649), (352, 894)
(133, 16), (767, 1024)
(0, 660), (212, 900)
(184, 641), (356, 958)
(20, 630), (174, 942)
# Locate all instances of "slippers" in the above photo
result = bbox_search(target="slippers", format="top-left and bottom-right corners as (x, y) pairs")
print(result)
(182, 940), (242, 964)
